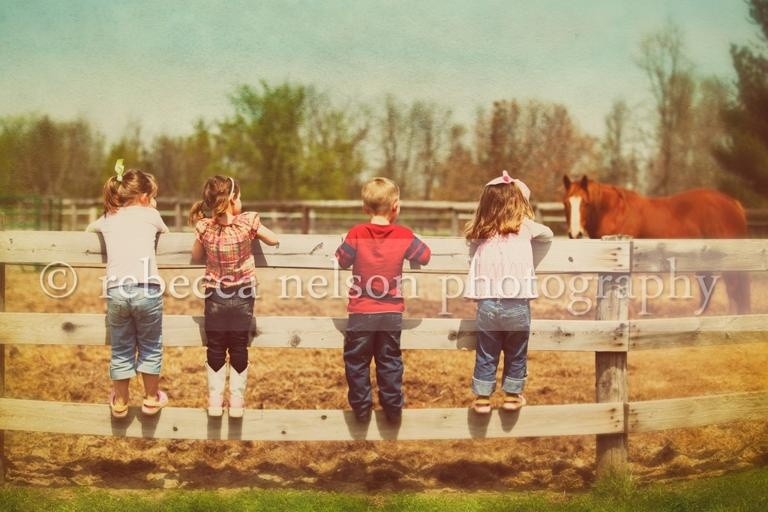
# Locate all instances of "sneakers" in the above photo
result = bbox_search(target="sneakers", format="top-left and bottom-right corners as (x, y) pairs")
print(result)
(356, 408), (372, 420)
(377, 392), (402, 422)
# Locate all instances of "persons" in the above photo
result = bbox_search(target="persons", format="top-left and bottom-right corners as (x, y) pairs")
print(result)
(334, 177), (431, 421)
(187, 174), (278, 420)
(83, 157), (170, 419)
(464, 170), (553, 416)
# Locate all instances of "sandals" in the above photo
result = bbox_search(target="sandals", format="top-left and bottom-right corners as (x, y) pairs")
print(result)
(500, 394), (527, 410)
(472, 396), (492, 414)
(109, 390), (130, 418)
(140, 390), (170, 415)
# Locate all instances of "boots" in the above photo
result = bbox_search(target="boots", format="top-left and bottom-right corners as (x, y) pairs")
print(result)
(204, 360), (228, 417)
(227, 361), (250, 418)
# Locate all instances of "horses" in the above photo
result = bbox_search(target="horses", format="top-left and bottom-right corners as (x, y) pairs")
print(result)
(562, 172), (752, 317)
